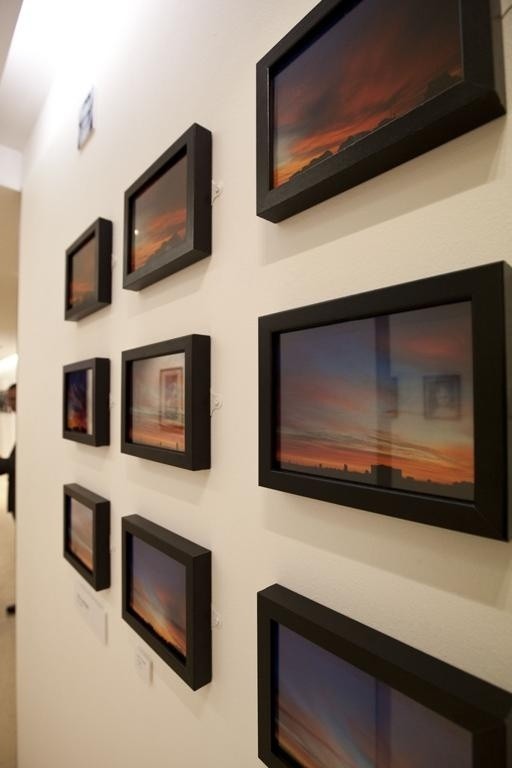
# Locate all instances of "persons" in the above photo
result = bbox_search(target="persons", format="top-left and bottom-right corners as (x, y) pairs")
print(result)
(0, 384), (16, 614)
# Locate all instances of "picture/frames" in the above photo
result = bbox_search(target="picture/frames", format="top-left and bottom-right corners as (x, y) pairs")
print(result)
(258, 260), (511, 541)
(257, 583), (512, 768)
(121, 514), (212, 691)
(121, 334), (211, 471)
(255, 0), (506, 223)
(124, 122), (212, 290)
(63, 483), (110, 591)
(64, 217), (112, 321)
(62, 357), (109, 446)
